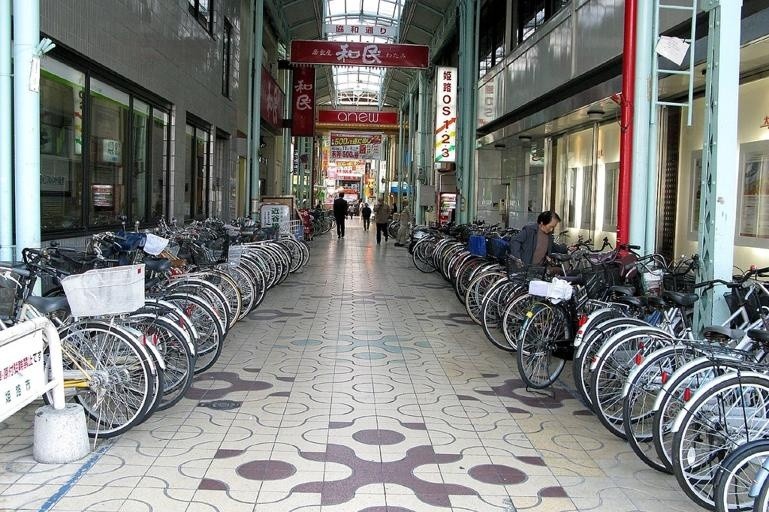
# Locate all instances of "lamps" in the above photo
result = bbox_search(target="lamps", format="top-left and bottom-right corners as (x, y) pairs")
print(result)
(519, 136), (532, 142)
(587, 110), (605, 120)
(495, 144), (505, 150)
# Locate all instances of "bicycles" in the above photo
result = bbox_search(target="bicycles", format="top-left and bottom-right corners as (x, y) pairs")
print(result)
(308, 208), (337, 236)
(384, 218), (400, 239)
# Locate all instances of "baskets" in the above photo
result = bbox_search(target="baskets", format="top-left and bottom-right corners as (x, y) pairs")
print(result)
(583, 262), (695, 327)
(723, 282), (769, 327)
(0, 250), (146, 316)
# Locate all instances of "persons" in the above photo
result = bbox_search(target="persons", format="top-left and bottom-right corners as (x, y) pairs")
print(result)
(347, 201), (371, 232)
(316, 201), (325, 211)
(333, 192), (348, 239)
(511, 210), (577, 342)
(373, 197), (390, 248)
